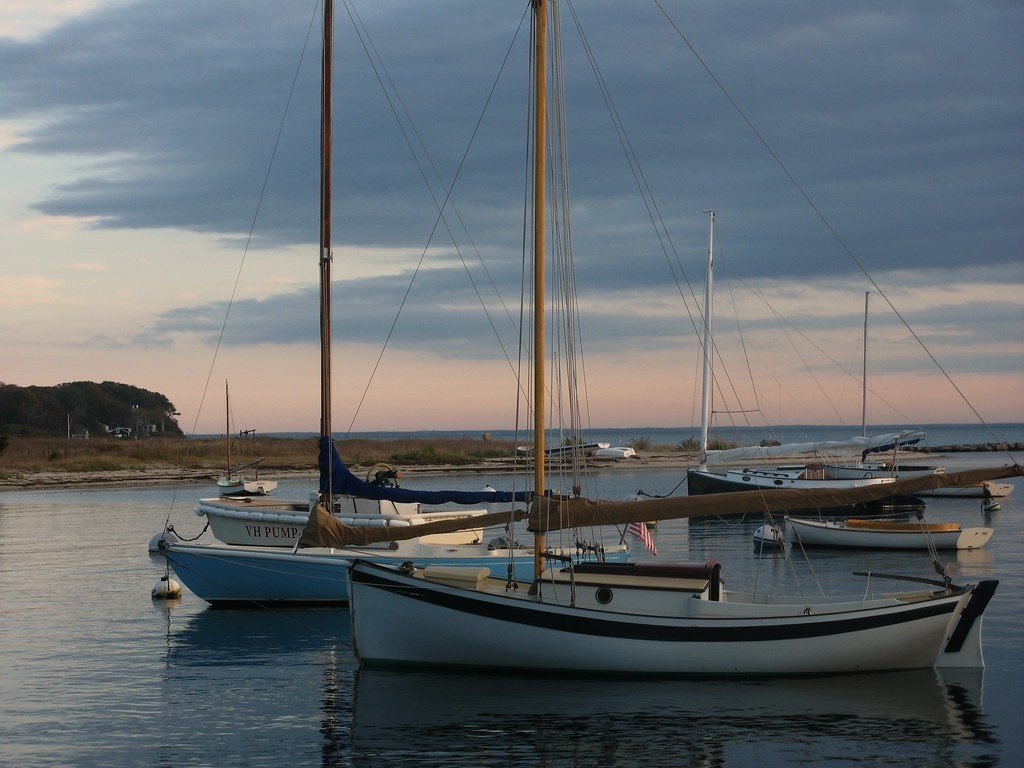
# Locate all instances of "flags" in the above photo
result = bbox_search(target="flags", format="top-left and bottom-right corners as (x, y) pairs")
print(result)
(626, 521), (660, 559)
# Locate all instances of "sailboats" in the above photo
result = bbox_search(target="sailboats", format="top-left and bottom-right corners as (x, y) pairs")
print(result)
(144, 0), (632, 604)
(679, 207), (897, 517)
(167, 600), (355, 670)
(347, 666), (1000, 767)
(211, 380), (279, 497)
(347, 0), (998, 674)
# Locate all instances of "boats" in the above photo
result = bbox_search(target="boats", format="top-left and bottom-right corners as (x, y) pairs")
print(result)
(774, 463), (1015, 498)
(514, 441), (639, 458)
(784, 514), (993, 552)
